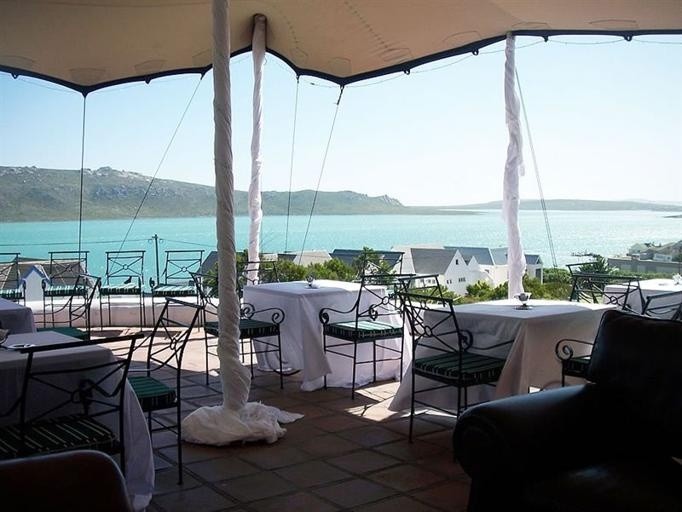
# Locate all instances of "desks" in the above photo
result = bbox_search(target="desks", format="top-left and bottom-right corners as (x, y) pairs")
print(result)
(244, 279), (387, 393)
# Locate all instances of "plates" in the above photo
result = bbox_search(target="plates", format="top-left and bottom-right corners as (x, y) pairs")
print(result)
(7, 343), (37, 349)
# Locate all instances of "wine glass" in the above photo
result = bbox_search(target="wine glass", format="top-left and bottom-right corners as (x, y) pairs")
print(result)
(513, 291), (534, 310)
(304, 274), (318, 289)
